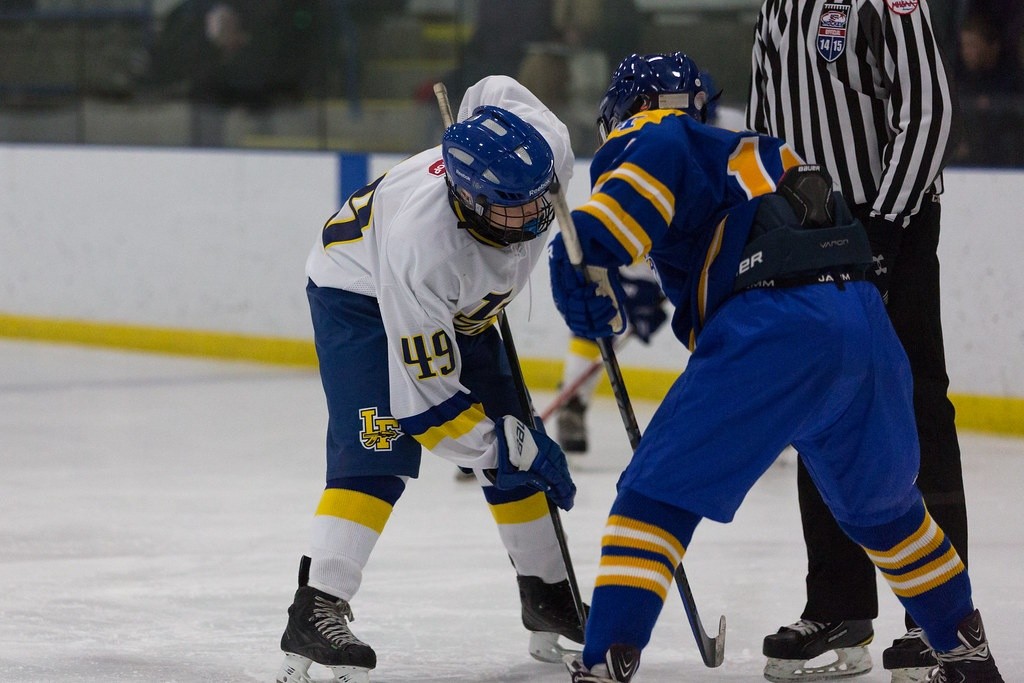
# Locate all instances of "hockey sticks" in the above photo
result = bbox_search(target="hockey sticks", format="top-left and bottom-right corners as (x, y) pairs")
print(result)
(548, 182), (727, 671)
(537, 331), (633, 425)
(433, 80), (586, 631)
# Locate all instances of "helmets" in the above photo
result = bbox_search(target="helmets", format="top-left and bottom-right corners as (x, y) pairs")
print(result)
(441, 105), (559, 247)
(596, 49), (709, 146)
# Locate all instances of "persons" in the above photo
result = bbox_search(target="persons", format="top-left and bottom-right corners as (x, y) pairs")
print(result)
(273, 74), (591, 683)
(743, 0), (968, 683)
(959, 14), (1024, 169)
(547, 50), (1003, 683)
(465, 0), (636, 105)
(166, 0), (324, 105)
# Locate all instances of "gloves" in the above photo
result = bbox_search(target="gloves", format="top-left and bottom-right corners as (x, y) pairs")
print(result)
(483, 413), (577, 511)
(547, 229), (626, 339)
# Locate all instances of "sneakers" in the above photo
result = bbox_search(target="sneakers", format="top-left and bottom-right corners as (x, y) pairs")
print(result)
(762, 616), (874, 682)
(566, 645), (640, 683)
(509, 553), (592, 663)
(924, 608), (1004, 683)
(276, 554), (377, 683)
(558, 393), (588, 455)
(882, 609), (938, 683)
